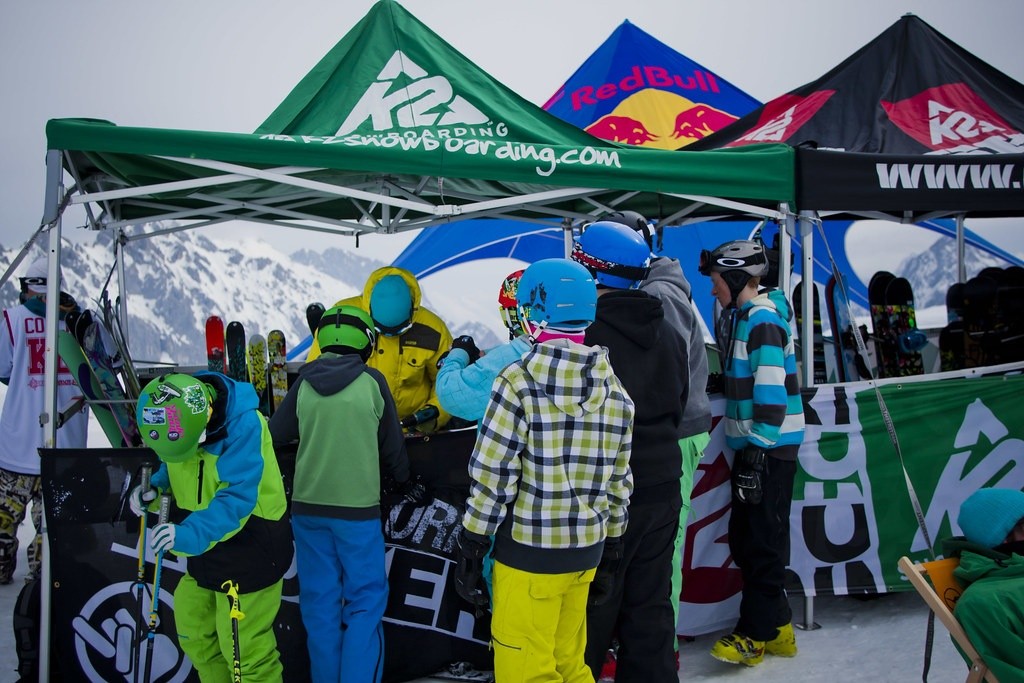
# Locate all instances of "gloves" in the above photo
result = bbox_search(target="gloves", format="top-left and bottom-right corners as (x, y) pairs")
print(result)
(457, 527), (493, 606)
(436, 334), (480, 370)
(151, 523), (176, 553)
(588, 536), (624, 606)
(395, 480), (415, 495)
(129, 484), (157, 517)
(731, 447), (766, 504)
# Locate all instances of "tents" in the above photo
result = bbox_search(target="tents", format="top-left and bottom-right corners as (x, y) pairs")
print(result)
(1, 0), (1024, 683)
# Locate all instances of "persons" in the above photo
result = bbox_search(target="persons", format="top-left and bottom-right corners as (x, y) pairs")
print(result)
(129, 369), (294, 682)
(597, 209), (711, 683)
(306, 267), (453, 435)
(267, 304), (405, 682)
(698, 239), (806, 668)
(0, 258), (124, 588)
(954, 487), (1024, 683)
(435, 270), (533, 421)
(571, 220), (691, 683)
(459, 258), (636, 683)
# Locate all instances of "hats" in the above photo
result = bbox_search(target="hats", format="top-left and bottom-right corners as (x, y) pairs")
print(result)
(26, 258), (63, 293)
(957, 487), (1024, 548)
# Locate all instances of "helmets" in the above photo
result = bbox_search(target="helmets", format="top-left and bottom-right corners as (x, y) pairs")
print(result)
(136, 372), (210, 463)
(369, 275), (413, 337)
(596, 210), (655, 252)
(515, 258), (598, 332)
(317, 305), (375, 364)
(699, 240), (769, 297)
(573, 221), (651, 290)
(498, 270), (525, 308)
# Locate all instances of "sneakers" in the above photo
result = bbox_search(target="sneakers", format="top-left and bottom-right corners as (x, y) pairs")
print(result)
(709, 633), (765, 666)
(766, 622), (797, 657)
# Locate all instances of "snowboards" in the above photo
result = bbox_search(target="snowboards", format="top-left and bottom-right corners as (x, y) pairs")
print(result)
(939, 266), (1024, 372)
(885, 277), (928, 378)
(868, 271), (900, 379)
(824, 272), (872, 382)
(793, 280), (827, 384)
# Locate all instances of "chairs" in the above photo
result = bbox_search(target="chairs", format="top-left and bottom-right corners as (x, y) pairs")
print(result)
(897, 556), (1001, 683)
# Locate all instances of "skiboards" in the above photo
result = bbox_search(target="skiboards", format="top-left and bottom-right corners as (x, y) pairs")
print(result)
(247, 334), (271, 419)
(205, 316), (227, 373)
(58, 290), (144, 448)
(226, 321), (247, 382)
(268, 330), (288, 412)
